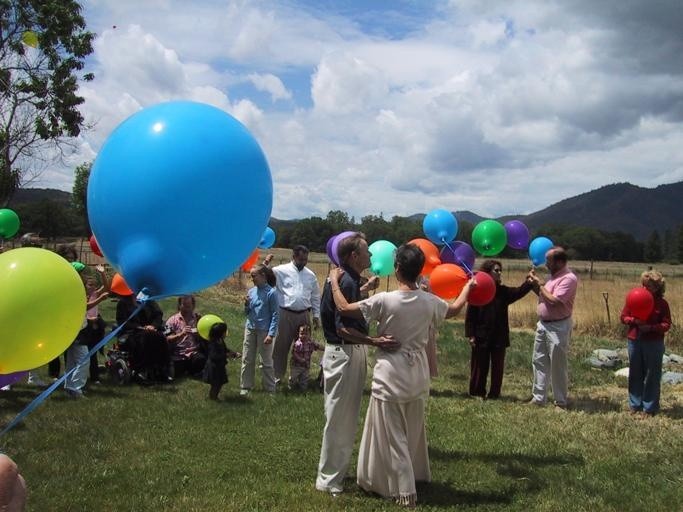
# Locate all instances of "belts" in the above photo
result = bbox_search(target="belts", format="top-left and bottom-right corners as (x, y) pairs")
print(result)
(540, 316), (572, 324)
(326, 340), (368, 345)
(276, 307), (314, 314)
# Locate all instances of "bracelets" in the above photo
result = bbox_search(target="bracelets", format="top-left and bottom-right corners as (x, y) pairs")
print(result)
(333, 288), (337, 290)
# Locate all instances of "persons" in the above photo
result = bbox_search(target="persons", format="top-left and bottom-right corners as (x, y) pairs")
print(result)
(85, 264), (110, 385)
(465, 260), (535, 401)
(261, 245), (321, 392)
(65, 261), (91, 402)
(620, 270), (672, 419)
(203, 323), (242, 400)
(49, 244), (78, 384)
(22, 233), (48, 387)
(241, 264), (280, 397)
(116, 294), (175, 383)
(329, 244), (477, 506)
(529, 247), (577, 410)
(315, 234), (403, 497)
(330, 261), (377, 301)
(165, 296), (211, 381)
(288, 324), (325, 390)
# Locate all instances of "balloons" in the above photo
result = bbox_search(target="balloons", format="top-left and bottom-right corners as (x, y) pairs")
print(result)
(424, 209), (459, 245)
(333, 232), (359, 266)
(430, 264), (468, 299)
(88, 100), (274, 301)
(529, 237), (555, 267)
(1, 246), (87, 373)
(327, 235), (339, 268)
(505, 221), (530, 250)
(468, 271), (496, 306)
(243, 249), (259, 272)
(90, 235), (104, 257)
(408, 239), (442, 275)
(259, 227), (275, 250)
(367, 240), (399, 278)
(441, 241), (474, 275)
(21, 30), (38, 48)
(111, 271), (134, 296)
(2, 371), (29, 390)
(198, 314), (229, 340)
(472, 220), (508, 256)
(0, 209), (21, 241)
(627, 289), (655, 320)
